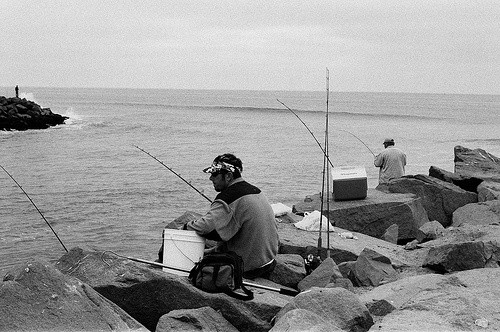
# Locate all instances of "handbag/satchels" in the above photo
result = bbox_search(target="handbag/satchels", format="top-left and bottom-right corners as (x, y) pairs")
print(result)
(188, 250), (255, 301)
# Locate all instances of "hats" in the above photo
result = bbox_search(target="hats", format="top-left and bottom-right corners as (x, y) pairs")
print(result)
(202, 153), (243, 173)
(383, 138), (395, 144)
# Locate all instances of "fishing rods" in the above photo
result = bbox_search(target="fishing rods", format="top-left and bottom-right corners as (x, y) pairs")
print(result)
(131, 144), (213, 203)
(338, 130), (375, 156)
(276, 98), (335, 167)
(317, 66), (330, 258)
(0, 164), (69, 252)
(124, 257), (298, 296)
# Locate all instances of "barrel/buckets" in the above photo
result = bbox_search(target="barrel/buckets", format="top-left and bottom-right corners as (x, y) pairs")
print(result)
(162, 228), (206, 277)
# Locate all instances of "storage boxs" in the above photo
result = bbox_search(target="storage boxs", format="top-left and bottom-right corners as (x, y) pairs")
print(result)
(331, 166), (368, 200)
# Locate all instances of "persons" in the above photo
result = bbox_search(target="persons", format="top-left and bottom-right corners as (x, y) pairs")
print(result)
(374, 137), (406, 185)
(15, 85), (19, 97)
(178, 154), (279, 279)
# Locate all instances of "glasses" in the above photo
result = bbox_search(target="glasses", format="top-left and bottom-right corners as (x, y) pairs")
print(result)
(211, 172), (221, 177)
